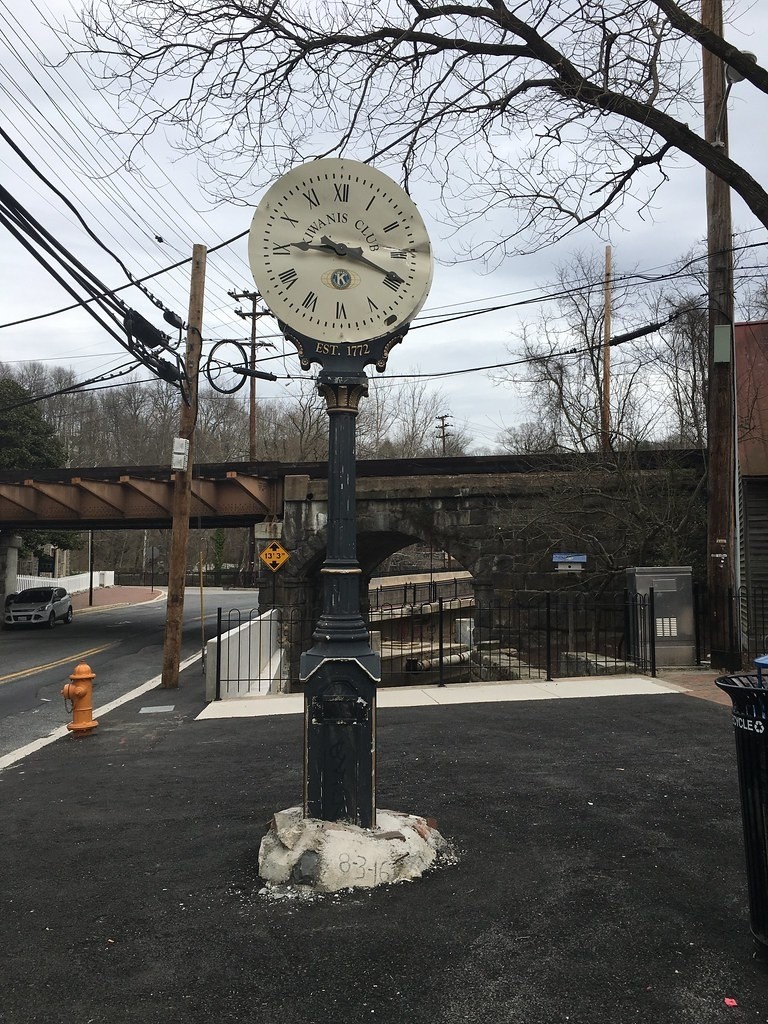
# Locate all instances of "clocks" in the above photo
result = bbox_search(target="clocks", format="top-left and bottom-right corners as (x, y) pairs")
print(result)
(248, 158), (435, 344)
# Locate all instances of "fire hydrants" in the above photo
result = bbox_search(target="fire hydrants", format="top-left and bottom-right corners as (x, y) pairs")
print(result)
(60, 662), (99, 737)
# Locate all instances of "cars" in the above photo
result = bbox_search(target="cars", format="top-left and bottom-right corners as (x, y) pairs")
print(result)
(3, 586), (73, 628)
